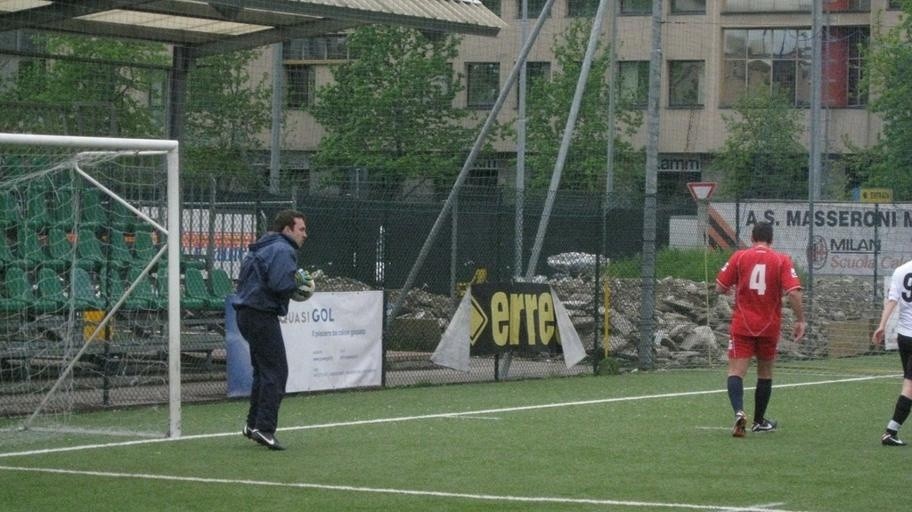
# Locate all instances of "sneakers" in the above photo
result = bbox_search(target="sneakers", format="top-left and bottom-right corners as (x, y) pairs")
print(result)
(880, 431), (908, 447)
(751, 419), (778, 434)
(730, 409), (747, 438)
(251, 428), (286, 451)
(242, 424), (253, 440)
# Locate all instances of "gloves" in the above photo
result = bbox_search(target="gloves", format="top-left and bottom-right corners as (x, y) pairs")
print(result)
(294, 267), (313, 291)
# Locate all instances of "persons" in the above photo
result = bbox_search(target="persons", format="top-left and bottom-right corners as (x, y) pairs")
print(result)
(713, 221), (807, 437)
(231, 208), (314, 450)
(871, 260), (912, 446)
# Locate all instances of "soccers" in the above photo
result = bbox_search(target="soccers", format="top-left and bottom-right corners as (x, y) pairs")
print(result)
(291, 275), (316, 302)
